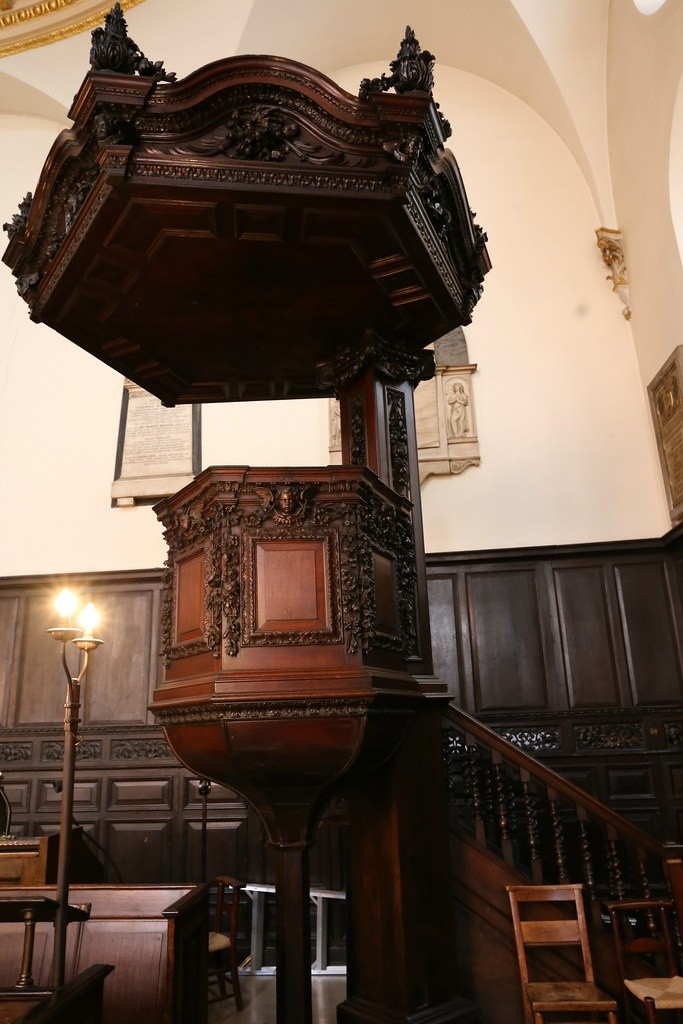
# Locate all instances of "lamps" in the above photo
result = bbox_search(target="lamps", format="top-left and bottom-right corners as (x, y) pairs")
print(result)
(46, 586), (104, 994)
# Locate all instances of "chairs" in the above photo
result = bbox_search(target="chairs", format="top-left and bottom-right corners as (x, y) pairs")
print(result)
(505, 884), (615, 1024)
(204, 876), (245, 1011)
(608, 900), (682, 1024)
